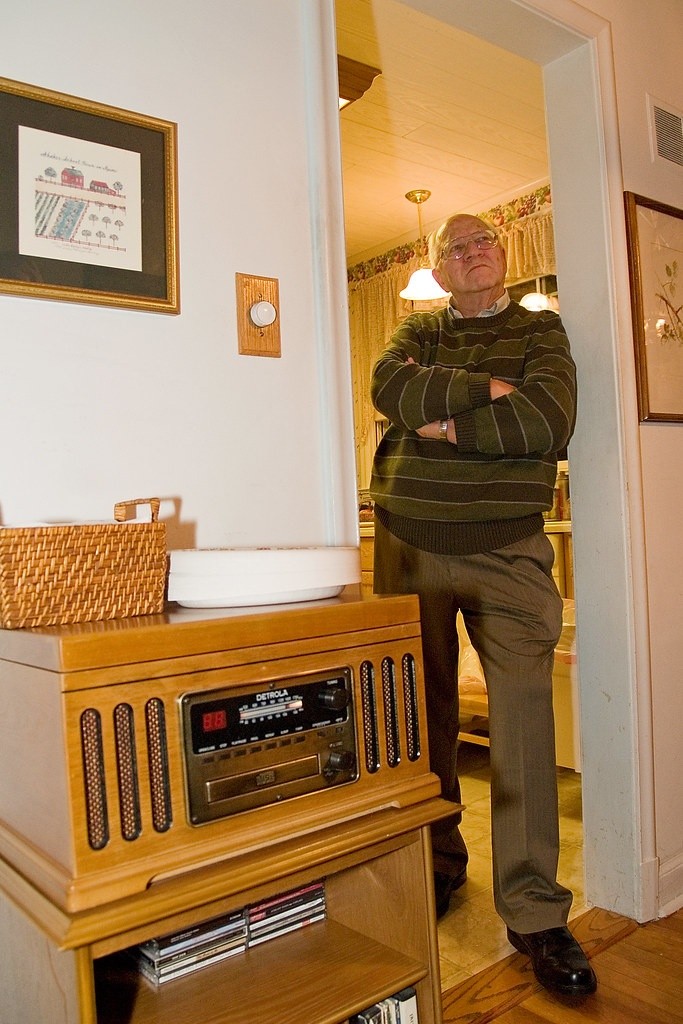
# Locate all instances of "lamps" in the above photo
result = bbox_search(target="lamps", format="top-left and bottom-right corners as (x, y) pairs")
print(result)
(399, 189), (451, 301)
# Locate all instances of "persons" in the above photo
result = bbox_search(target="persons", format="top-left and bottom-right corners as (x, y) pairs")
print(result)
(370, 213), (596, 1006)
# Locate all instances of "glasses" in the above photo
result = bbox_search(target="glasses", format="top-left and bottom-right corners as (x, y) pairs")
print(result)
(436, 229), (499, 268)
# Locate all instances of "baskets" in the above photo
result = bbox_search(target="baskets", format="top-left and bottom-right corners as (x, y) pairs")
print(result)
(0, 497), (169, 630)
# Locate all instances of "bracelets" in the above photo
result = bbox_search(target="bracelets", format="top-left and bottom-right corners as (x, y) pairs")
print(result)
(440, 416), (451, 442)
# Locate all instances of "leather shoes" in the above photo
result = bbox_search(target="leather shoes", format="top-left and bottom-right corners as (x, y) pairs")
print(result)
(506, 926), (598, 996)
(434, 865), (468, 921)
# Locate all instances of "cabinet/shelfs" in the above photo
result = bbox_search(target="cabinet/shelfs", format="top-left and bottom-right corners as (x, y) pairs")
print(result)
(0, 797), (467, 1024)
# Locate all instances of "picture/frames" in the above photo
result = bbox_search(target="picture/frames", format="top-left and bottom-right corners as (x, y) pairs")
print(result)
(0, 76), (181, 315)
(623, 189), (683, 424)
